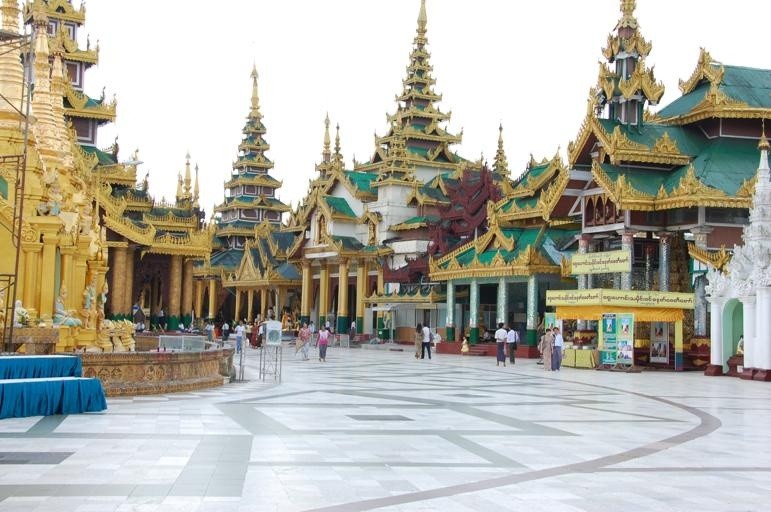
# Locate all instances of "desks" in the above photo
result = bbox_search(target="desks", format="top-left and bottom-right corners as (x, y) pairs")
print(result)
(1, 354), (107, 420)
(561, 349), (595, 369)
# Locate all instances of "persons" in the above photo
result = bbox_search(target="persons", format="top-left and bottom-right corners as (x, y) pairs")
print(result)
(459, 336), (469, 357)
(81, 277), (96, 330)
(421, 321), (434, 360)
(538, 328), (551, 372)
(591, 331), (598, 351)
(53, 286), (81, 328)
(382, 307), (388, 329)
(11, 299), (28, 329)
(551, 327), (564, 372)
(506, 324), (519, 366)
(97, 280), (109, 324)
(493, 322), (507, 367)
(158, 306), (356, 363)
(383, 310), (391, 326)
(735, 335), (743, 355)
(608, 324), (667, 359)
(479, 328), (492, 344)
(414, 323), (424, 360)
(133, 296), (146, 332)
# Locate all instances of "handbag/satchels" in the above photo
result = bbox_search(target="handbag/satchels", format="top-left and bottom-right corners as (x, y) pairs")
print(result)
(513, 342), (518, 351)
(430, 333), (434, 341)
